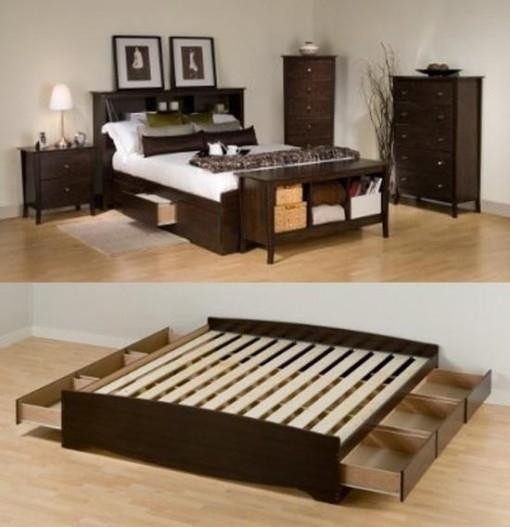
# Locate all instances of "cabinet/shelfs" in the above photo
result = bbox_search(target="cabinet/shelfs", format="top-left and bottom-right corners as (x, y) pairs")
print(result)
(281, 54), (337, 149)
(14, 311), (497, 507)
(389, 75), (485, 218)
(234, 160), (393, 264)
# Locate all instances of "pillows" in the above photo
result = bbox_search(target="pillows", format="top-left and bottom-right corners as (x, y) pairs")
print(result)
(101, 112), (259, 157)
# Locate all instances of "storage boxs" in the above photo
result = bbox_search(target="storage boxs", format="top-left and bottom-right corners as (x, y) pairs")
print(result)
(273, 185), (381, 234)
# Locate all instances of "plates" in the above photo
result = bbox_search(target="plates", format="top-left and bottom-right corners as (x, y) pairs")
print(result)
(415, 68), (463, 77)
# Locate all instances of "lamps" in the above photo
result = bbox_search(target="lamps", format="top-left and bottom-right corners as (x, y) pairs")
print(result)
(49, 85), (73, 147)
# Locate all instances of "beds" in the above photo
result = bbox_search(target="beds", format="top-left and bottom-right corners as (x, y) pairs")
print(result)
(90, 87), (359, 254)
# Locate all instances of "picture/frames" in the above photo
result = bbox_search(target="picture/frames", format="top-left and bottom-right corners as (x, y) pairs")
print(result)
(169, 36), (217, 88)
(112, 35), (165, 89)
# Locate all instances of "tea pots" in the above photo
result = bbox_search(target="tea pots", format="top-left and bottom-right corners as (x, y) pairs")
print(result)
(207, 140), (228, 157)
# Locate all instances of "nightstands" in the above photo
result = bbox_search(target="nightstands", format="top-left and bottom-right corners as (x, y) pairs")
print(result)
(17, 144), (96, 224)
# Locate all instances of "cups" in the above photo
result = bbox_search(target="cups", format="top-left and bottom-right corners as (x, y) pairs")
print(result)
(226, 145), (241, 155)
